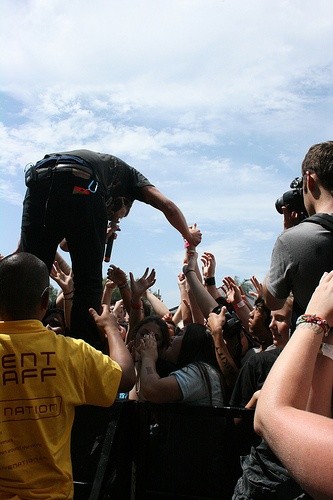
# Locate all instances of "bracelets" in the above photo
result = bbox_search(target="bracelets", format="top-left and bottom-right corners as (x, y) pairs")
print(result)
(296, 314), (331, 337)
(204, 277), (215, 286)
(184, 242), (190, 248)
(234, 300), (246, 311)
(186, 269), (195, 277)
(63, 291), (74, 300)
(118, 282), (129, 289)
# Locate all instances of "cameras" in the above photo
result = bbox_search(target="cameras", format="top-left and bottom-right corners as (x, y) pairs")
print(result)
(275, 176), (305, 214)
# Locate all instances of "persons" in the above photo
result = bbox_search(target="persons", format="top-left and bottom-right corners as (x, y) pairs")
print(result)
(254, 269), (333, 499)
(0, 251), (135, 500)
(232, 141), (333, 500)
(0, 251), (333, 408)
(19, 149), (203, 357)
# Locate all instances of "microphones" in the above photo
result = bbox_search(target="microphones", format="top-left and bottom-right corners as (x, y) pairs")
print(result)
(105, 221), (117, 262)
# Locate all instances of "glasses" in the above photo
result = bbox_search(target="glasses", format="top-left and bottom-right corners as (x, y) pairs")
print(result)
(141, 329), (161, 341)
(41, 285), (54, 296)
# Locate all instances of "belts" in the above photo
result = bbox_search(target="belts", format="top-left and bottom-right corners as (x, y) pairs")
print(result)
(36, 164), (93, 176)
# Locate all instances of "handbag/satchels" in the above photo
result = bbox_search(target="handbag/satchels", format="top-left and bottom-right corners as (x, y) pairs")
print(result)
(232, 443), (309, 500)
